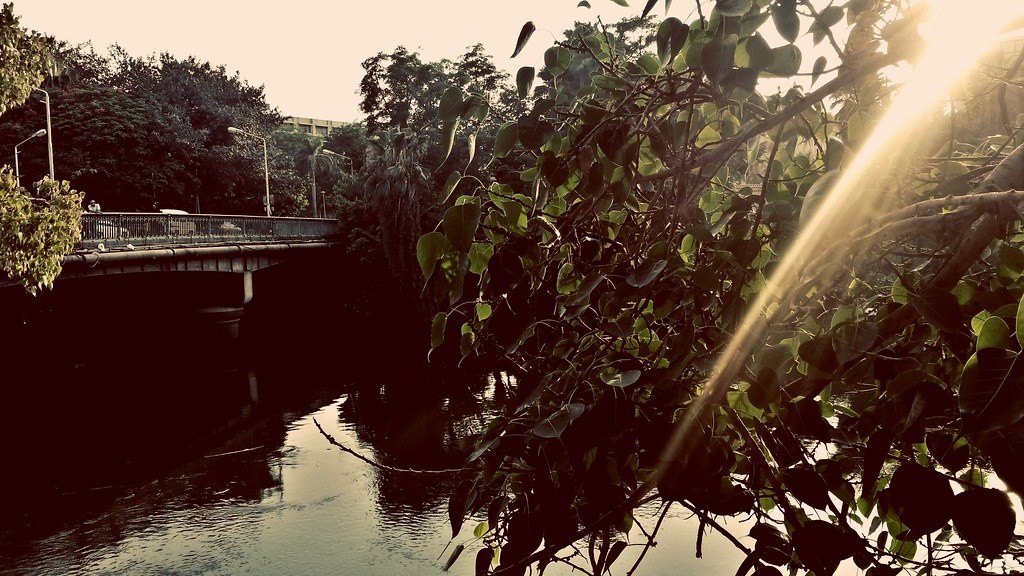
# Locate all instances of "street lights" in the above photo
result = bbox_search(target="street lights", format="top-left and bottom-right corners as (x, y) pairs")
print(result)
(14, 129), (47, 188)
(322, 149), (353, 176)
(228, 127), (270, 216)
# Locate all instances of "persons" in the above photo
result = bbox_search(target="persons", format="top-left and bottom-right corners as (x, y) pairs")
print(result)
(82, 200), (101, 240)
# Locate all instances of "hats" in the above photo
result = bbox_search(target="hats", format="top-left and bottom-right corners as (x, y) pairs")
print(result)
(90, 200), (95, 202)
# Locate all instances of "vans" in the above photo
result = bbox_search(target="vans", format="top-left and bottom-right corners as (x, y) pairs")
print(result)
(159, 208), (197, 236)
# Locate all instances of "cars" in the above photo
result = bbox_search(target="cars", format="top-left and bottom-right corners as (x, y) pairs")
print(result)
(203, 221), (241, 235)
(97, 220), (129, 238)
